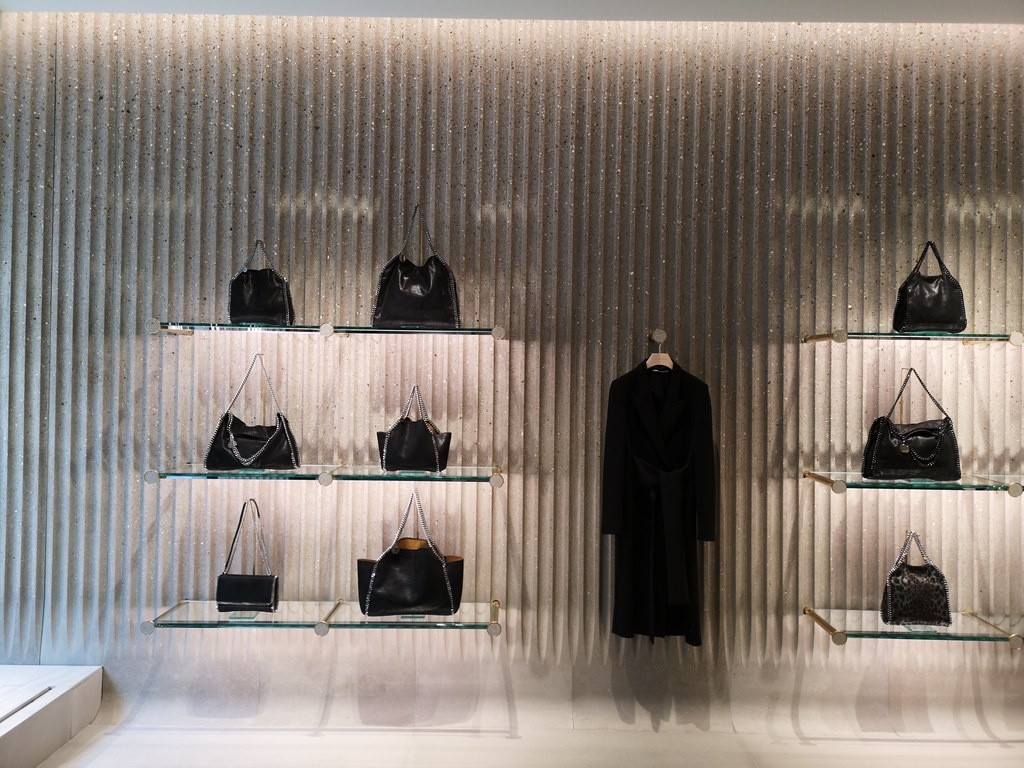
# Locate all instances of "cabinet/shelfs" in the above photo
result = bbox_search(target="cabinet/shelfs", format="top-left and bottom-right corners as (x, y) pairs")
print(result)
(802, 330), (1024, 650)
(144, 317), (505, 638)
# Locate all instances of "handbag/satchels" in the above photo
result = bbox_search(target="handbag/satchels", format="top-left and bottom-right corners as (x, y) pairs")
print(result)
(861, 367), (962, 481)
(371, 205), (461, 330)
(227, 240), (295, 326)
(201, 354), (301, 469)
(215, 498), (280, 613)
(880, 532), (952, 626)
(892, 241), (967, 333)
(357, 488), (463, 617)
(377, 384), (452, 471)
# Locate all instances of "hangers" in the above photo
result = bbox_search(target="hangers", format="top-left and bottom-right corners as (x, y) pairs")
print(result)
(645, 328), (673, 373)
